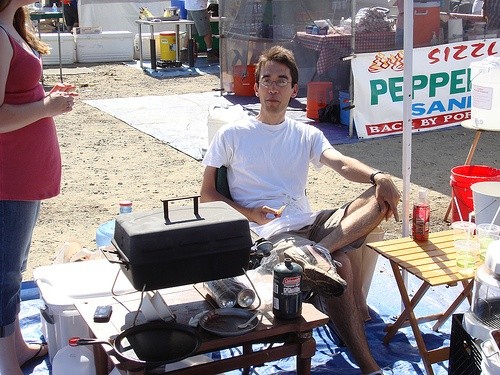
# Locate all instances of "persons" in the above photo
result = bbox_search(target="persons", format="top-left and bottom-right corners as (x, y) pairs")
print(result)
(0, 0), (79, 375)
(181, 0), (219, 62)
(200, 45), (401, 375)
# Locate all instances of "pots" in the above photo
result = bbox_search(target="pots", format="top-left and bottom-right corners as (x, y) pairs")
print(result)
(76, 320), (202, 365)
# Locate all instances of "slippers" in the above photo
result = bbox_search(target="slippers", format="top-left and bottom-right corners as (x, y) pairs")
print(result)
(21, 342), (48, 368)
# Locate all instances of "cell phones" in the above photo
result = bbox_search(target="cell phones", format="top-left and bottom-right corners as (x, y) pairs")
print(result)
(93, 305), (113, 322)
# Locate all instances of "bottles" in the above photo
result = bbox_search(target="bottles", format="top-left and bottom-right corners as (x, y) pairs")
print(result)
(412, 190), (430, 244)
(52, 3), (57, 12)
(273, 258), (304, 320)
(96, 201), (132, 251)
(340, 16), (345, 27)
(51, 336), (96, 375)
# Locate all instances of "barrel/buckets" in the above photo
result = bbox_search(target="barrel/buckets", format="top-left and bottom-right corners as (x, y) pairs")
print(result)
(234, 65), (256, 96)
(469, 61), (500, 130)
(160, 31), (176, 61)
(450, 165), (500, 235)
(306, 81), (334, 120)
(338, 89), (349, 125)
(413, 1), (440, 46)
(470, 181), (500, 229)
(478, 340), (500, 375)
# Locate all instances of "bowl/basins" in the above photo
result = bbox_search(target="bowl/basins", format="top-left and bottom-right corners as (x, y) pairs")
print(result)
(140, 291), (175, 322)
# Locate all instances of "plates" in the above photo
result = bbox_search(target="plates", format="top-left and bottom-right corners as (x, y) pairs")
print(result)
(199, 306), (259, 336)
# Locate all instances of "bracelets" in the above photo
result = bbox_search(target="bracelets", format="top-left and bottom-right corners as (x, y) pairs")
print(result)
(369, 171), (385, 186)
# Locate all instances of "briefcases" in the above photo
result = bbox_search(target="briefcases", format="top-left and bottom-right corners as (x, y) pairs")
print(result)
(100, 195), (251, 291)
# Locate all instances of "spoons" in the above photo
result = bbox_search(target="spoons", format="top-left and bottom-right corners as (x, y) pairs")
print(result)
(238, 311), (259, 329)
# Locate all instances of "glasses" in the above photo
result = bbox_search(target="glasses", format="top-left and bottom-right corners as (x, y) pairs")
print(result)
(259, 78), (293, 87)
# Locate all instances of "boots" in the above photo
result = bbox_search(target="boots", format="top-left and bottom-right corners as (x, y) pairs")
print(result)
(180, 49), (196, 64)
(206, 48), (219, 62)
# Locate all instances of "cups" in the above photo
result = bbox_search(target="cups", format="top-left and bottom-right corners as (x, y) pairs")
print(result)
(453, 219), (476, 254)
(476, 223), (500, 260)
(454, 239), (480, 275)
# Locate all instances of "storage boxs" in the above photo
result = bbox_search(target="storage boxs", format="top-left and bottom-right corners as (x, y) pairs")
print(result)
(32, 259), (138, 364)
(135, 32), (161, 59)
(447, 312), (483, 375)
(34, 32), (77, 64)
(75, 29), (132, 63)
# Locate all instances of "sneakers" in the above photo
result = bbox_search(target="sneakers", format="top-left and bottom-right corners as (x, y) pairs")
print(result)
(367, 367), (394, 375)
(284, 243), (348, 298)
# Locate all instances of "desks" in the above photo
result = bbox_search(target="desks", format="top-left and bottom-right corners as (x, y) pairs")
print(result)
(74, 271), (328, 375)
(364, 224), (486, 375)
(135, 18), (194, 67)
(294, 27), (396, 82)
(30, 10), (63, 83)
